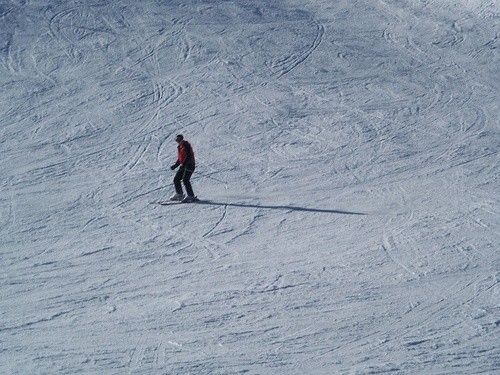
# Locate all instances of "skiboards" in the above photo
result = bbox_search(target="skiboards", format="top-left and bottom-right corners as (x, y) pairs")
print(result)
(147, 195), (200, 205)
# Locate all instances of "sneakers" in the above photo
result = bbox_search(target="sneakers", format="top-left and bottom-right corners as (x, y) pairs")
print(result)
(182, 195), (194, 202)
(170, 194), (183, 200)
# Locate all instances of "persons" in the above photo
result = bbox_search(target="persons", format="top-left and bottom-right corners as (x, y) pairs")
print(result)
(169, 135), (195, 203)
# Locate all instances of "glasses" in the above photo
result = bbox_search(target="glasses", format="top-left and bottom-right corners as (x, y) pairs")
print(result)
(176, 138), (182, 142)
